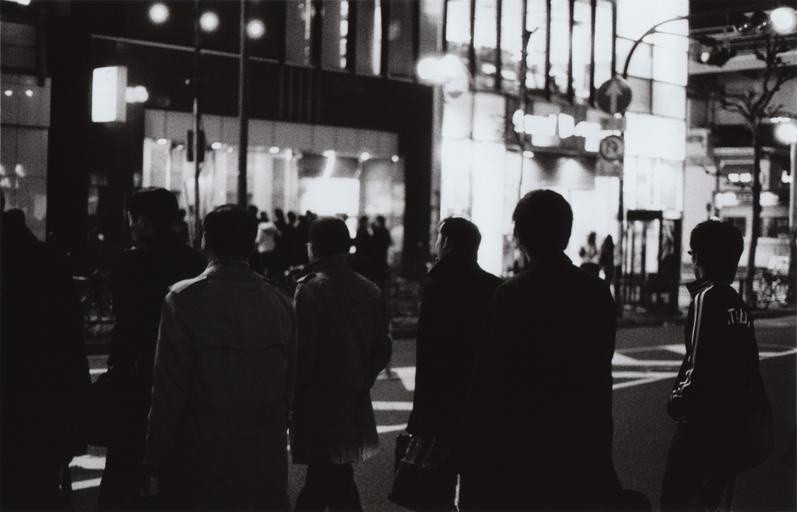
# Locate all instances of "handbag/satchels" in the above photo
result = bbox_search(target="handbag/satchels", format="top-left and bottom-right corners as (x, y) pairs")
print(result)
(736, 374), (775, 475)
(388, 434), (460, 511)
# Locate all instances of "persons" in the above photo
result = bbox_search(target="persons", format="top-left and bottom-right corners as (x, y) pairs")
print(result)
(385, 216), (508, 511)
(140, 202), (296, 511)
(578, 232), (597, 265)
(248, 205), (392, 285)
(656, 218), (777, 511)
(0, 190), (84, 510)
(285, 216), (392, 510)
(94, 187), (209, 510)
(456, 189), (654, 510)
(595, 234), (614, 282)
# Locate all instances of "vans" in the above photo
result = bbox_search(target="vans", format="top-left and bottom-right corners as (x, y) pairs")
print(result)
(689, 24), (741, 42)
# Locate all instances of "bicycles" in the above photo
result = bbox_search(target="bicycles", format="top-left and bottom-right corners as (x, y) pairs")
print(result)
(752, 262), (793, 311)
